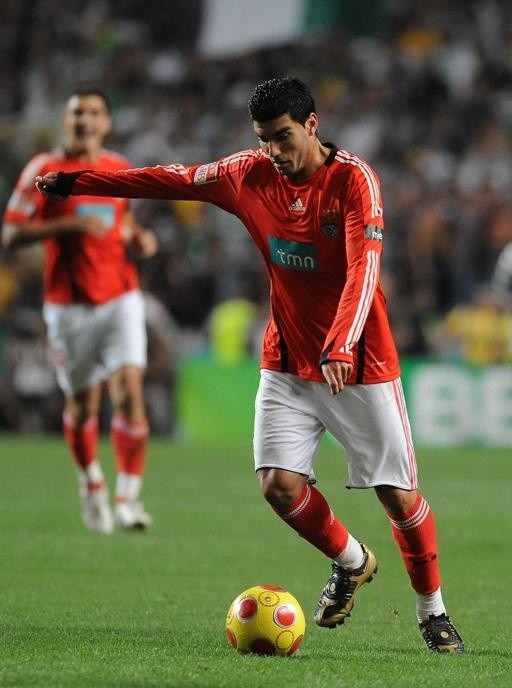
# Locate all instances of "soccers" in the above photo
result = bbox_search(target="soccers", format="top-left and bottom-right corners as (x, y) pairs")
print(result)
(226, 585), (305, 656)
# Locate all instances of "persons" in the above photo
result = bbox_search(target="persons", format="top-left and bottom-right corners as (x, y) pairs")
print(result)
(0, 86), (161, 536)
(33, 70), (468, 655)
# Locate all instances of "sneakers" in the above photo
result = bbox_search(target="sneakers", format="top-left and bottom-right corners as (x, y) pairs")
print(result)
(81, 490), (112, 534)
(313, 539), (378, 629)
(117, 500), (147, 528)
(418, 612), (465, 655)
